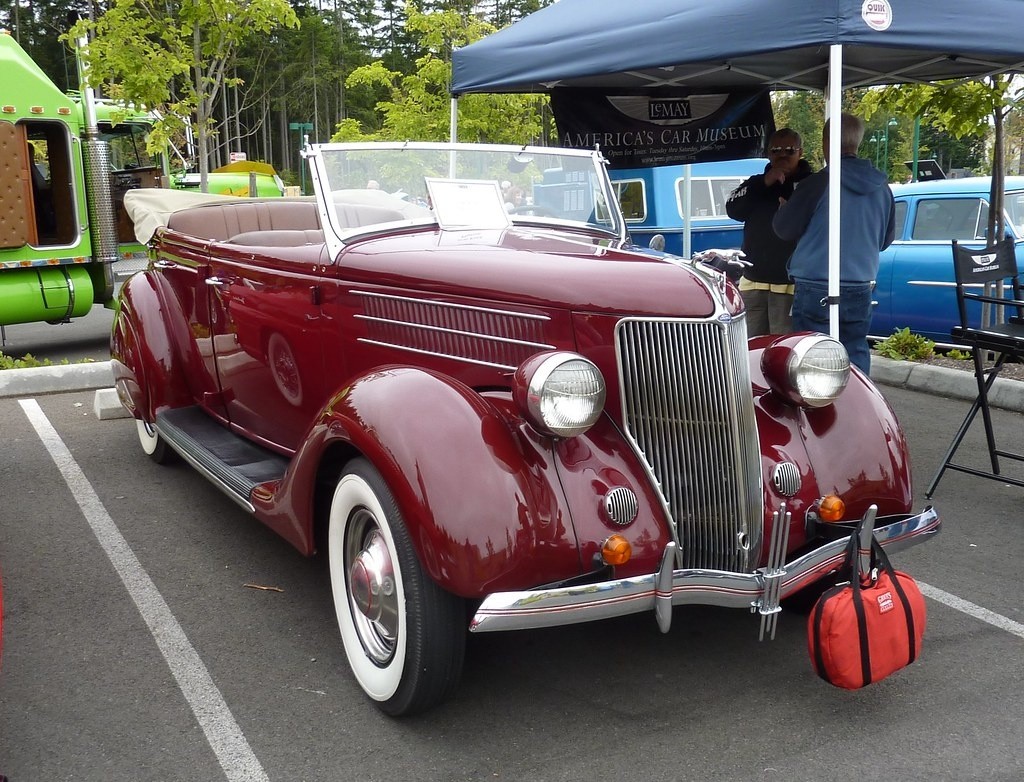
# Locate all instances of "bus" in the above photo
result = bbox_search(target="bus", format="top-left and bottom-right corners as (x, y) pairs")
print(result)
(0, 28), (288, 338)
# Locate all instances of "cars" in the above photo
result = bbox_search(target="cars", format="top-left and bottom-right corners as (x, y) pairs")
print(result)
(108, 132), (942, 722)
(856, 175), (1024, 363)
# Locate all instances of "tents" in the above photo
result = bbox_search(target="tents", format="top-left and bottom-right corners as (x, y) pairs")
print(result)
(447, 1), (1024, 343)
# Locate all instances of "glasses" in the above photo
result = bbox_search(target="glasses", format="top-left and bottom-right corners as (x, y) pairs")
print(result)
(768, 145), (802, 156)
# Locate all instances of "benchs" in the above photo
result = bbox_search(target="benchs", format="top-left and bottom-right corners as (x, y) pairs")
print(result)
(168, 198), (404, 249)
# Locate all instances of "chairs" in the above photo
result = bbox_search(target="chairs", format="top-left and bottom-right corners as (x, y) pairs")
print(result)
(926, 235), (1024, 499)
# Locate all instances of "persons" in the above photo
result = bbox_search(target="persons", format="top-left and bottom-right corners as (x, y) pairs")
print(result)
(774, 113), (896, 375)
(725, 127), (814, 339)
